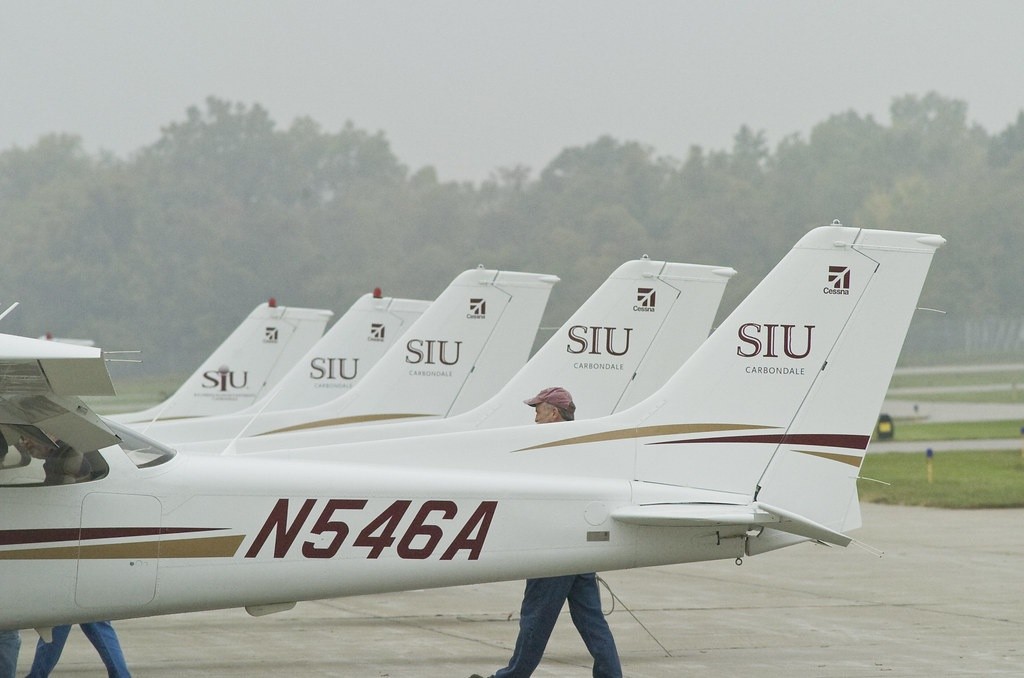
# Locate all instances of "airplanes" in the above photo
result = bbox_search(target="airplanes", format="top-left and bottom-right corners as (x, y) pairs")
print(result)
(0, 223), (948, 644)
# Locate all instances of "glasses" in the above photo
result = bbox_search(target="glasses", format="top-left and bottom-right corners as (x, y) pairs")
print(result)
(18, 436), (25, 448)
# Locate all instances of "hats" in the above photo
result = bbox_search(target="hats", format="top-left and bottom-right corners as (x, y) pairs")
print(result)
(524, 387), (575, 413)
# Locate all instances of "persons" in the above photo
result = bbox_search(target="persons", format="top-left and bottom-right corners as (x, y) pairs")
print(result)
(469, 388), (623, 678)
(0, 428), (132, 678)
